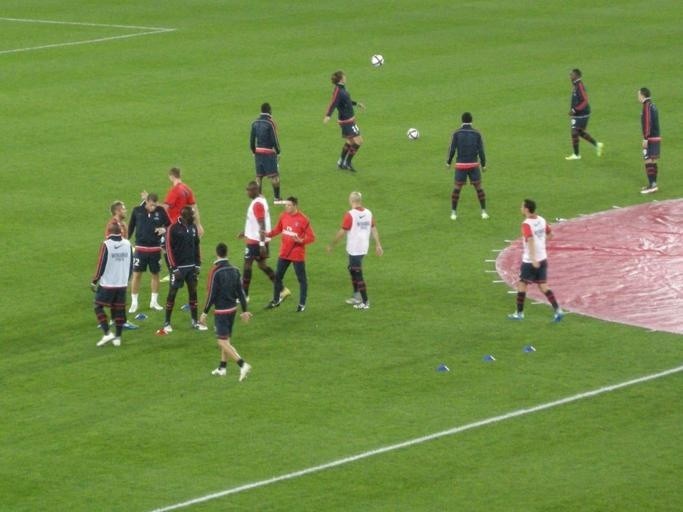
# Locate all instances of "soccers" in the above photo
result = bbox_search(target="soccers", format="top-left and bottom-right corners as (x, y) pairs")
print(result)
(372, 55), (384, 67)
(408, 128), (419, 139)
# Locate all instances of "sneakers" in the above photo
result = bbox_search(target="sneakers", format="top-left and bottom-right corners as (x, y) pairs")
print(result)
(565, 153), (581, 160)
(157, 323), (172, 332)
(239, 362), (251, 381)
(112, 338), (121, 347)
(353, 300), (369, 309)
(507, 310), (524, 320)
(597, 143), (604, 157)
(129, 304), (137, 312)
(97, 332), (115, 346)
(150, 302), (163, 310)
(552, 310), (564, 322)
(211, 367), (227, 376)
(281, 287), (292, 299)
(450, 213), (457, 221)
(236, 297), (250, 304)
(268, 301), (280, 308)
(296, 304), (305, 312)
(160, 275), (170, 282)
(122, 321), (137, 329)
(338, 158), (356, 173)
(481, 212), (488, 218)
(192, 320), (208, 330)
(640, 184), (658, 194)
(345, 296), (362, 304)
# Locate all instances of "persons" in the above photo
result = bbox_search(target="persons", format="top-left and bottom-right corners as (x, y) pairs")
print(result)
(637, 87), (662, 194)
(506, 198), (564, 322)
(327, 191), (384, 310)
(564, 68), (604, 160)
(445, 111), (491, 220)
(249, 102), (285, 204)
(322, 70), (367, 173)
